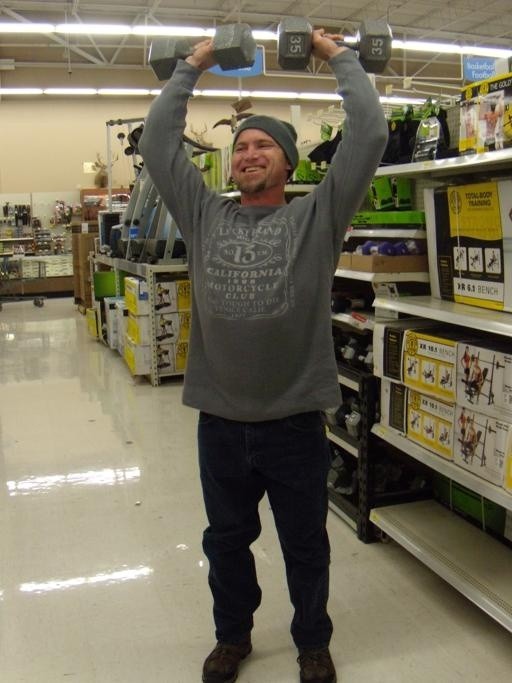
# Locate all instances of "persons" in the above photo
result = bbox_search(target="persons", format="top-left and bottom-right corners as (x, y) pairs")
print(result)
(459, 345), (471, 392)
(462, 106), (475, 152)
(457, 406), (466, 451)
(463, 418), (478, 447)
(470, 357), (484, 389)
(476, 94), (504, 151)
(139, 26), (389, 682)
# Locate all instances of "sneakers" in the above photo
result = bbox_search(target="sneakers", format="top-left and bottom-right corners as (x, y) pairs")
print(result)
(201, 630), (254, 683)
(295, 639), (336, 683)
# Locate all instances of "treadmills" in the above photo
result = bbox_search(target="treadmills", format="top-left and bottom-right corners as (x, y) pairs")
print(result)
(157, 350), (171, 369)
(111, 123), (220, 264)
(155, 289), (171, 311)
(156, 320), (174, 341)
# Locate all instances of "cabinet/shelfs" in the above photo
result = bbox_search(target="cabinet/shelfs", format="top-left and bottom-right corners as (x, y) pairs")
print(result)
(86, 251), (188, 387)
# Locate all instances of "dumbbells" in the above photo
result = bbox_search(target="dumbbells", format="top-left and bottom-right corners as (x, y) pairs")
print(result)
(148, 23), (258, 81)
(276, 17), (393, 74)
(332, 293), (373, 313)
(327, 398), (361, 440)
(332, 327), (373, 372)
(362, 240), (408, 257)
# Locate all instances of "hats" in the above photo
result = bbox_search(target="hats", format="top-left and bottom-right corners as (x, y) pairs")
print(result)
(231, 114), (299, 181)
